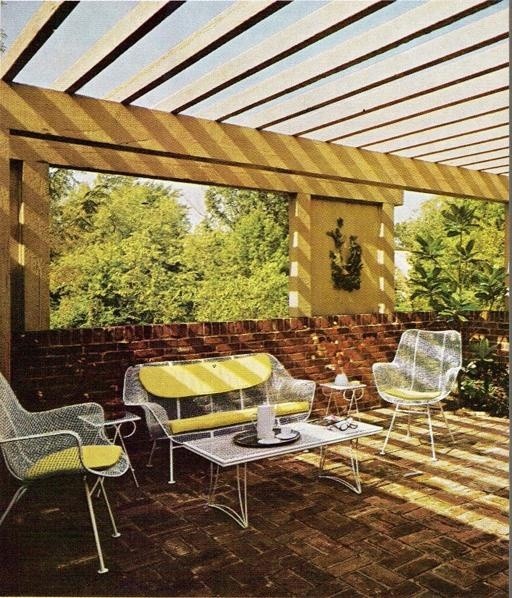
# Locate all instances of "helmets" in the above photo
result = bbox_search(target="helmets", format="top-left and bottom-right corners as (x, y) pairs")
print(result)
(256, 438), (280, 444)
(276, 431), (296, 439)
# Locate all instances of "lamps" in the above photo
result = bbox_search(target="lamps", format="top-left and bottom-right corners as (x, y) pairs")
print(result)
(0, 374), (131, 576)
(372, 329), (462, 462)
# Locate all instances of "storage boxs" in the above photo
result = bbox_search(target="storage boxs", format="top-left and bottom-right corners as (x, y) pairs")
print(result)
(122, 352), (316, 484)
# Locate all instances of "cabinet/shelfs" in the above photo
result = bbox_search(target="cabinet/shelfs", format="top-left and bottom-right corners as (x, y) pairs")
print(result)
(335, 366), (349, 385)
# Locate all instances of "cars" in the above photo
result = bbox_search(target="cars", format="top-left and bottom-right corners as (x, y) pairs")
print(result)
(251, 405), (275, 439)
(352, 380), (360, 386)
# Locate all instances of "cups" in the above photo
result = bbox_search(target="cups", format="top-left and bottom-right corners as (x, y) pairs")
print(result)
(334, 423), (357, 431)
